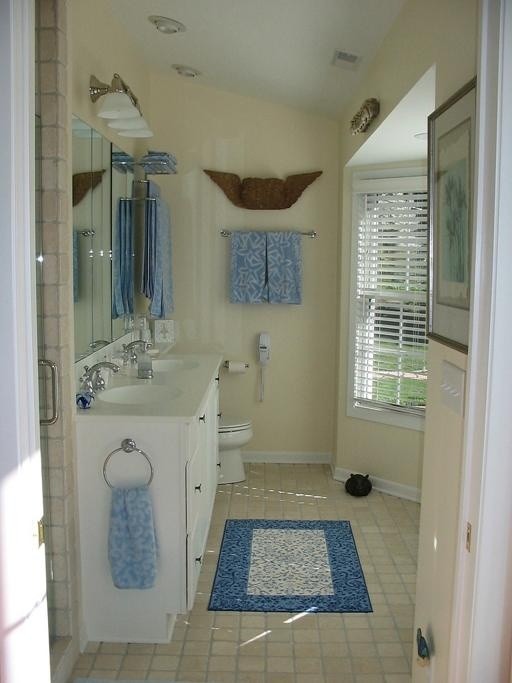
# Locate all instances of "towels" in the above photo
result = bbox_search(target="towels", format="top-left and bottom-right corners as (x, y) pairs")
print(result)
(74, 229), (79, 304)
(141, 149), (178, 176)
(112, 198), (134, 319)
(228, 231), (302, 306)
(142, 180), (175, 320)
(112, 151), (137, 172)
(106, 485), (158, 590)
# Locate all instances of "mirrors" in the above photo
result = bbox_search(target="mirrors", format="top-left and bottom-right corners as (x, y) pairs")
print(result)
(72, 112), (142, 364)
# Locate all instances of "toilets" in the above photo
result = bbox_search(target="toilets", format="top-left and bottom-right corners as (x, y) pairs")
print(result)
(218, 415), (254, 485)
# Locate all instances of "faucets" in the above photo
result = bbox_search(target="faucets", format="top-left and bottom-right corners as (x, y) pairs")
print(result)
(80, 361), (121, 387)
(123, 340), (148, 353)
(89, 340), (111, 348)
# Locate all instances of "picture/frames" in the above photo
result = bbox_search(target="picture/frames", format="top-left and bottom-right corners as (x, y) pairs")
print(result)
(425, 75), (477, 355)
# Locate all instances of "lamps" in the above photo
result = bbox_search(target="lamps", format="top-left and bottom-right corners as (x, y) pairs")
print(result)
(89, 71), (156, 139)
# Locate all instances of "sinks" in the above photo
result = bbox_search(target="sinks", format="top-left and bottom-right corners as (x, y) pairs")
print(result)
(135, 358), (200, 370)
(98, 385), (169, 405)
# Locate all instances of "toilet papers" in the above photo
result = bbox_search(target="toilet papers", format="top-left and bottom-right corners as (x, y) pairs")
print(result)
(228, 360), (246, 375)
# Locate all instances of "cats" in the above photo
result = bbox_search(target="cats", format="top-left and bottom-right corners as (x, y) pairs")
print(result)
(344, 473), (373, 497)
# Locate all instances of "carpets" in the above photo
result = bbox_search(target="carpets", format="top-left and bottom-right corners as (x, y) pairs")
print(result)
(206, 517), (374, 615)
